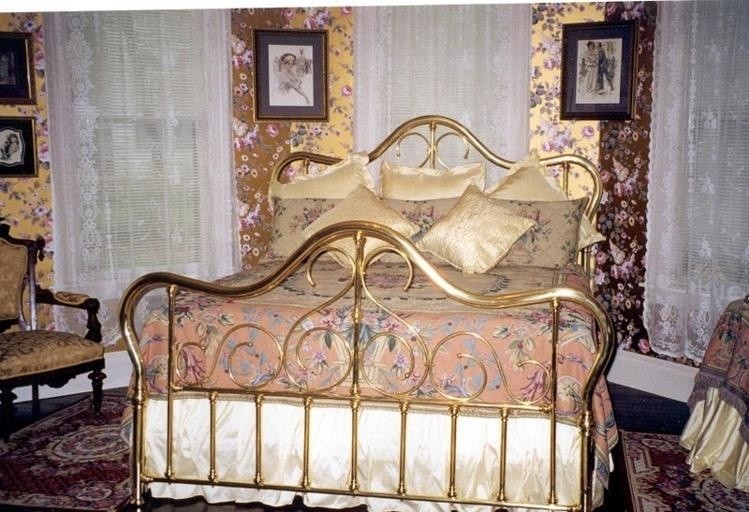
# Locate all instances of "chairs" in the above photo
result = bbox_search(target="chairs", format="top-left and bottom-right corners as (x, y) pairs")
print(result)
(1, 223), (106, 444)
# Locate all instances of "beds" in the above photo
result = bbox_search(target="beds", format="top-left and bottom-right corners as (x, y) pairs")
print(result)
(117, 113), (618, 512)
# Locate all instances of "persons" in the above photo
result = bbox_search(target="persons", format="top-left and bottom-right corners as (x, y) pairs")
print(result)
(579, 42), (600, 97)
(594, 42), (615, 96)
(4, 135), (19, 160)
(294, 56), (313, 107)
(270, 53), (311, 106)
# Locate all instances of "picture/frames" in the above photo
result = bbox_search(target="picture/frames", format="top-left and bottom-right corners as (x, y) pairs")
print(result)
(251, 27), (329, 123)
(559, 18), (640, 121)
(0, 31), (39, 179)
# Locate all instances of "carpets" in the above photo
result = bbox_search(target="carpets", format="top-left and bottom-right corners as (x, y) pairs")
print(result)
(621, 428), (749, 511)
(1, 391), (133, 512)
